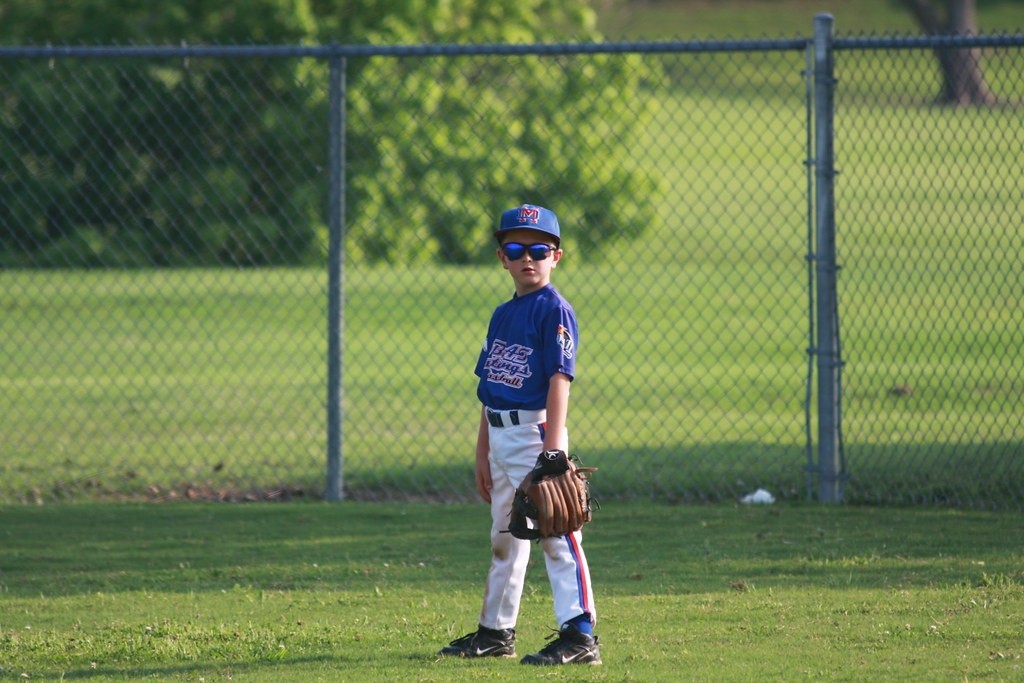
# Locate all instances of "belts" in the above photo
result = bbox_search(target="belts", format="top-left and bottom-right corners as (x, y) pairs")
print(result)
(487, 410), (520, 428)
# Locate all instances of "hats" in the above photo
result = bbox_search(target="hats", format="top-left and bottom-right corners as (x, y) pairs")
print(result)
(494, 204), (560, 241)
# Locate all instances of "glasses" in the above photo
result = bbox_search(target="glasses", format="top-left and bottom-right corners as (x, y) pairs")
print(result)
(502, 242), (557, 261)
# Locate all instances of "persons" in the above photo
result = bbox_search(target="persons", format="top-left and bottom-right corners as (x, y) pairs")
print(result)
(439, 204), (603, 668)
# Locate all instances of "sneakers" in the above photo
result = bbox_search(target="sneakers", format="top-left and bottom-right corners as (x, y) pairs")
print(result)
(519, 620), (603, 666)
(436, 623), (517, 659)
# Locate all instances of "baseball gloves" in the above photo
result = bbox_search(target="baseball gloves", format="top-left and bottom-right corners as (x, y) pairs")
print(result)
(508, 448), (594, 541)
